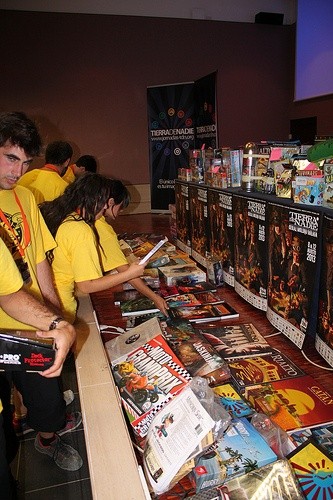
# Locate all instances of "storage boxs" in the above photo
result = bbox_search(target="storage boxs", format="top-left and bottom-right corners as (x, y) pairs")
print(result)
(157, 264), (206, 287)
(230, 150), (243, 187)
(178, 168), (192, 182)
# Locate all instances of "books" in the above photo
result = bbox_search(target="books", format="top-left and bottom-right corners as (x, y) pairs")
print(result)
(106, 231), (333, 500)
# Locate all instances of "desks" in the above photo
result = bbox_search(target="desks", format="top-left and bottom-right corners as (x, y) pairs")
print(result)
(73, 232), (331, 500)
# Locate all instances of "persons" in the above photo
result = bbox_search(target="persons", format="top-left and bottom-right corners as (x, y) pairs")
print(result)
(0, 111), (171, 472)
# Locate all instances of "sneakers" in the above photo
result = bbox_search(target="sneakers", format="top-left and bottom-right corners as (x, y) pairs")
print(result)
(34, 432), (83, 472)
(57, 411), (81, 436)
